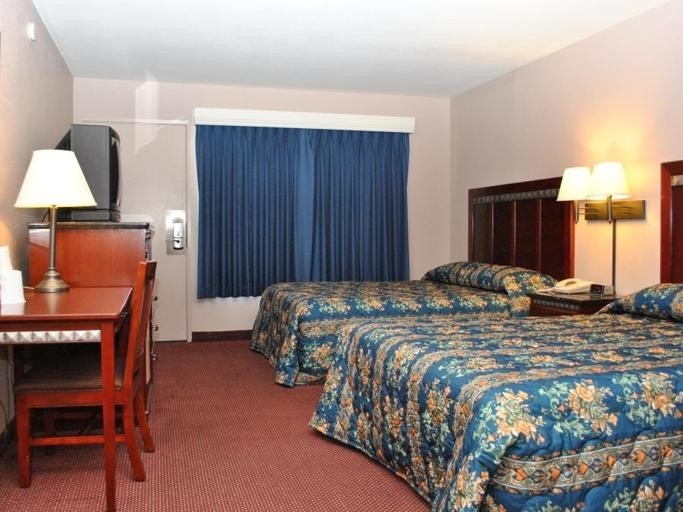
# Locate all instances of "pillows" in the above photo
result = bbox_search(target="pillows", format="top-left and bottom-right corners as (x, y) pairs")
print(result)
(422, 259), (557, 299)
(598, 282), (683, 322)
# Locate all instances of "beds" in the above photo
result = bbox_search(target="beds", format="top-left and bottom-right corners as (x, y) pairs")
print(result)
(250, 176), (576, 386)
(309, 161), (683, 511)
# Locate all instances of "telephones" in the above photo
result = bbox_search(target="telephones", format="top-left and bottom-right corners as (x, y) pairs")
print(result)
(549, 277), (593, 293)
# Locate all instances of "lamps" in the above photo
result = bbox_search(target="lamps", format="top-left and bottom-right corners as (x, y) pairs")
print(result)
(556, 165), (597, 223)
(588, 162), (631, 222)
(14, 148), (98, 293)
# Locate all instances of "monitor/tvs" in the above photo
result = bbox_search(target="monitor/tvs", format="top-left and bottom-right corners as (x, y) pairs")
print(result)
(49, 123), (122, 221)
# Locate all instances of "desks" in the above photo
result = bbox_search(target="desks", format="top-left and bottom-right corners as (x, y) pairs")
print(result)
(1, 285), (140, 512)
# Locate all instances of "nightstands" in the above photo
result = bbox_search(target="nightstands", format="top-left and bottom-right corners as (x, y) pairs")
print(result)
(525, 289), (619, 316)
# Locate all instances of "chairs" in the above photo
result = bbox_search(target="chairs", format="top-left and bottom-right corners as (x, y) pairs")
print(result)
(11, 257), (157, 489)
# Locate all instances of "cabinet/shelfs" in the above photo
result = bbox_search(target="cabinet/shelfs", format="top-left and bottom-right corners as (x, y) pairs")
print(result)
(21, 221), (154, 417)
